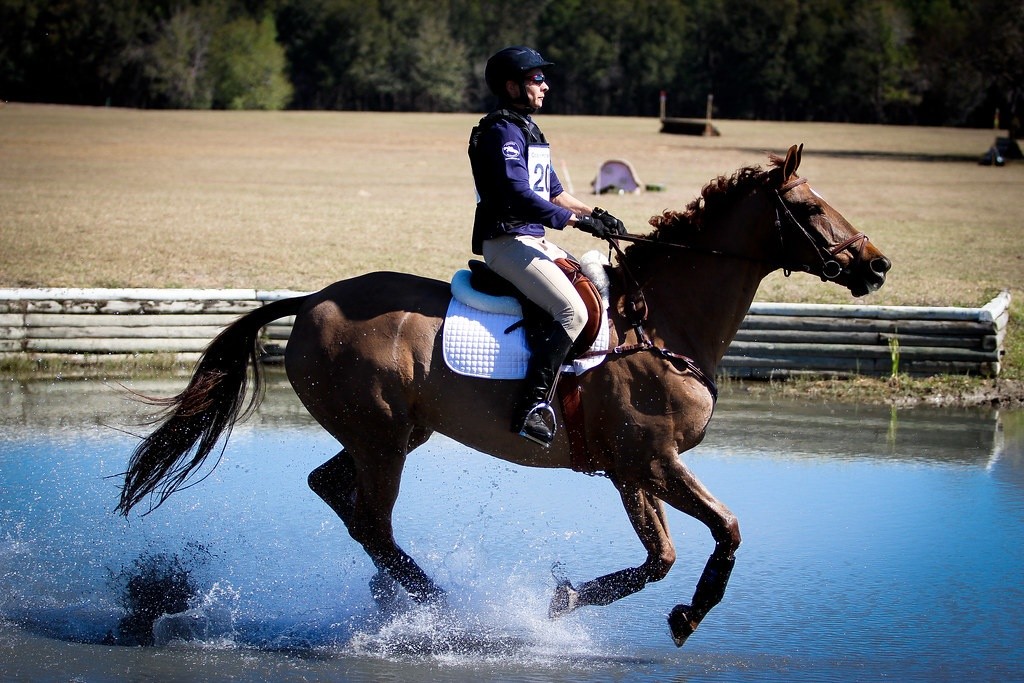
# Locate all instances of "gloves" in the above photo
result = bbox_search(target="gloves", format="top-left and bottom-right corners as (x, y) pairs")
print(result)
(573, 213), (611, 241)
(591, 204), (630, 236)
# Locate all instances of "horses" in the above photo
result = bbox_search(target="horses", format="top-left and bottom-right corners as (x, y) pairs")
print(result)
(101, 144), (891, 648)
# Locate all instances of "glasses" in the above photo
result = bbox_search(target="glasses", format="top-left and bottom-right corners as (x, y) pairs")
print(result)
(519, 73), (546, 84)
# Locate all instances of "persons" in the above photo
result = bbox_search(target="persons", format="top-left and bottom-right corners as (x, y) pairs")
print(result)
(466, 45), (630, 446)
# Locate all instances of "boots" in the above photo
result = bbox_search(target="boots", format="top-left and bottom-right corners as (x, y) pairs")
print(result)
(510, 321), (573, 443)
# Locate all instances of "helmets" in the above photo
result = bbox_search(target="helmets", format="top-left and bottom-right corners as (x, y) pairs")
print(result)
(484, 46), (555, 95)
